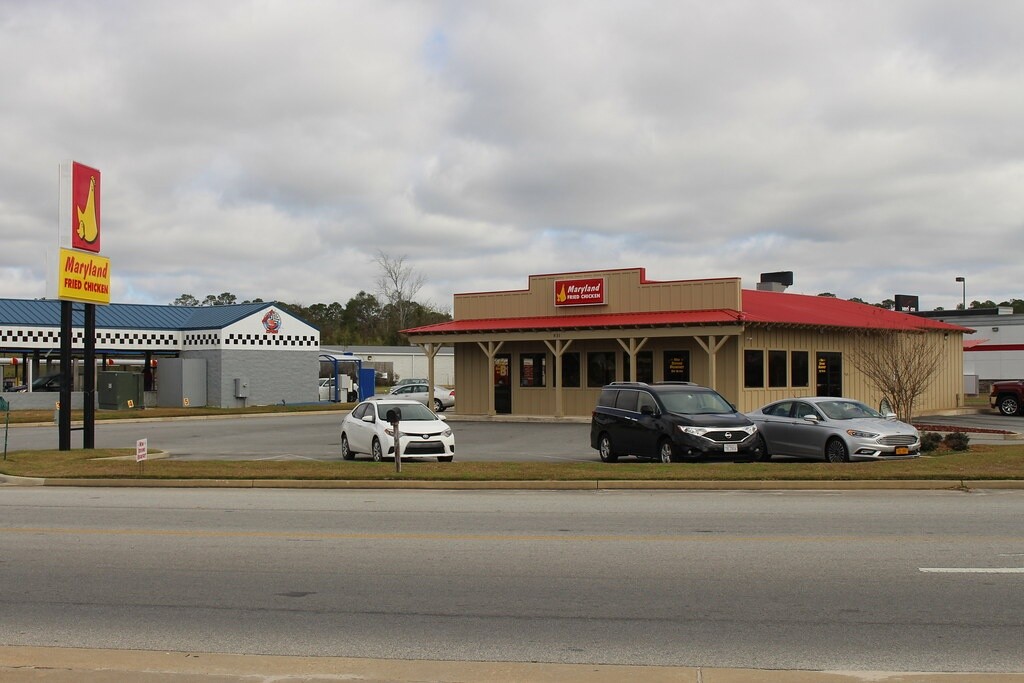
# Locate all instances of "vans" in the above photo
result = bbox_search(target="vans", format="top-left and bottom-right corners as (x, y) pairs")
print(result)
(591, 381), (759, 464)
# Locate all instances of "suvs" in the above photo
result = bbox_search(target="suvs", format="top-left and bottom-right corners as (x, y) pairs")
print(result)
(989, 381), (1024, 417)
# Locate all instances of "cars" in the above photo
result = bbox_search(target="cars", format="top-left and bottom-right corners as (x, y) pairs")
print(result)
(8, 373), (74, 392)
(390, 378), (429, 393)
(744, 397), (921, 463)
(365, 383), (455, 412)
(340, 399), (456, 462)
(319, 378), (358, 403)
